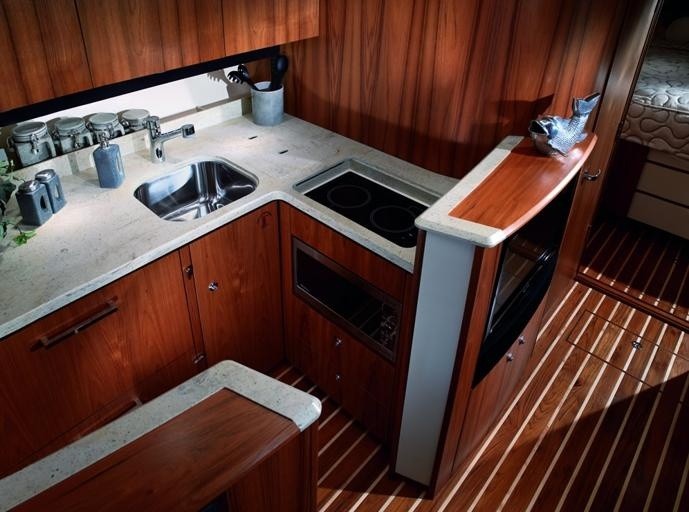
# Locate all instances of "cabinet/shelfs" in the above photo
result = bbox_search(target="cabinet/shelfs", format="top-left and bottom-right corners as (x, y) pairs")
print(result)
(452, 287), (552, 474)
(1, 0), (319, 112)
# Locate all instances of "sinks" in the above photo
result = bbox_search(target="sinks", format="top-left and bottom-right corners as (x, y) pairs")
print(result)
(133, 155), (259, 222)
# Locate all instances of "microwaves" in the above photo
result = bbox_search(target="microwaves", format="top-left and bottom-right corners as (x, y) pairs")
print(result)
(287, 236), (404, 364)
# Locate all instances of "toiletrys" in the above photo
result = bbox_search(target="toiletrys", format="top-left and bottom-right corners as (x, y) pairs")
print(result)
(92, 131), (125, 189)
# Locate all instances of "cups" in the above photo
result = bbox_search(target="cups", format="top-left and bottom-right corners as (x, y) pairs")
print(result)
(249, 81), (286, 126)
(49, 116), (94, 155)
(120, 108), (150, 133)
(85, 111), (126, 142)
(4, 120), (57, 168)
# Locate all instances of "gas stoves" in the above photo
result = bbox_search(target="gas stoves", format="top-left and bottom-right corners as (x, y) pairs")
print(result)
(291, 157), (444, 250)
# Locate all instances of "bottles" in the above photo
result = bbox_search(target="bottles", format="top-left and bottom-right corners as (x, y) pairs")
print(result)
(33, 168), (66, 213)
(14, 178), (53, 227)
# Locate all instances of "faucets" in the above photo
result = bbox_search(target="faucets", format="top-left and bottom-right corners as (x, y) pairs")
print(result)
(145, 116), (195, 163)
